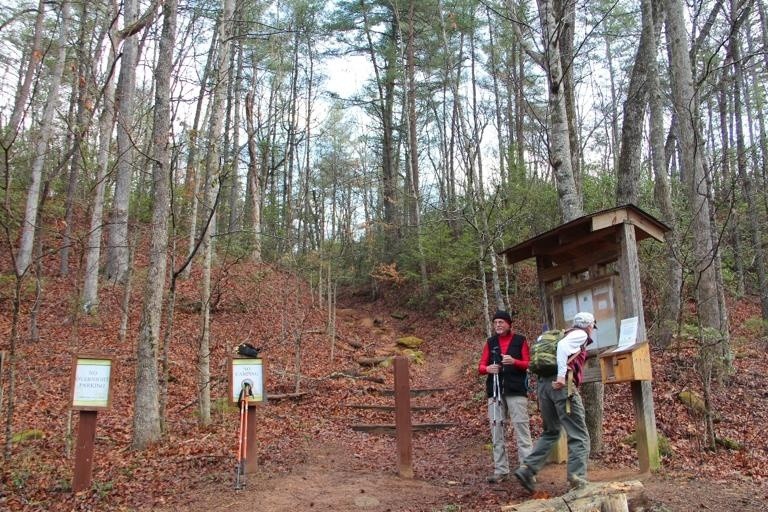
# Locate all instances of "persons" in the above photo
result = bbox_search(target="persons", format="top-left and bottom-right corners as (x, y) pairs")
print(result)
(513, 310), (597, 495)
(478, 307), (535, 483)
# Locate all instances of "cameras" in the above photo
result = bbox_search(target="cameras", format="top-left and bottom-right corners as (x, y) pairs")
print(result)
(491, 348), (502, 362)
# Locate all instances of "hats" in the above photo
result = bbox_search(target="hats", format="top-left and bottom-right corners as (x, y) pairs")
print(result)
(491, 310), (511, 324)
(573, 311), (597, 330)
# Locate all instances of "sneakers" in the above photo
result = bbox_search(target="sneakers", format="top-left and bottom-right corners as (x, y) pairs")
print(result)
(513, 466), (537, 493)
(572, 478), (594, 487)
(485, 473), (509, 483)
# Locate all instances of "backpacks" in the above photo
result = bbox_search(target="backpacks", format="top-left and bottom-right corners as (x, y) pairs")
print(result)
(527, 328), (588, 417)
